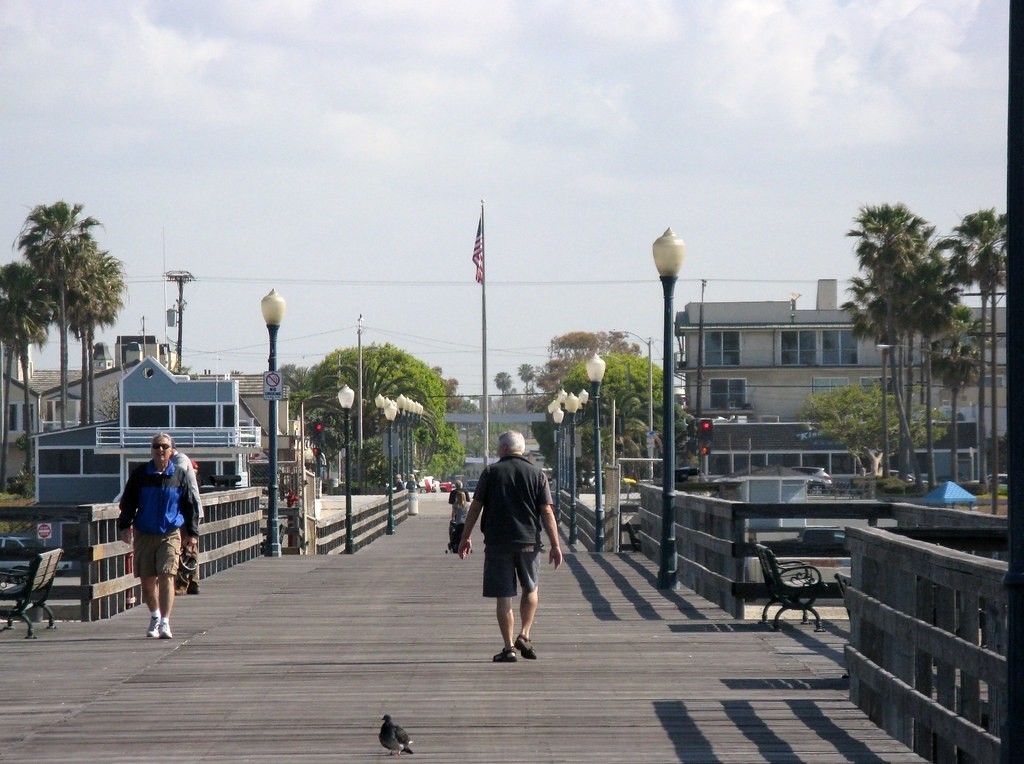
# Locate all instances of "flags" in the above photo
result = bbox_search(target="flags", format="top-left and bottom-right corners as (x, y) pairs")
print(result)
(472, 212), (484, 284)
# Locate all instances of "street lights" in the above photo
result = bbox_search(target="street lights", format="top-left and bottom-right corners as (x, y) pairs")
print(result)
(653, 228), (685, 589)
(385, 402), (397, 535)
(547, 388), (589, 544)
(586, 352), (606, 551)
(375, 394), (423, 484)
(338, 383), (354, 554)
(261, 288), (285, 558)
(875, 343), (981, 481)
(613, 332), (653, 479)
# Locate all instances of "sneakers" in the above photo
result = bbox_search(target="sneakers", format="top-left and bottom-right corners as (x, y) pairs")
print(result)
(147, 616), (160, 638)
(160, 622), (173, 638)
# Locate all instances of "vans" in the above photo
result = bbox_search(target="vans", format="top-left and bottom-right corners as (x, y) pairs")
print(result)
(417, 476), (434, 493)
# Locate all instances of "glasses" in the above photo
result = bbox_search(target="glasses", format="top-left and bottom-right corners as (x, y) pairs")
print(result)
(152, 443), (171, 449)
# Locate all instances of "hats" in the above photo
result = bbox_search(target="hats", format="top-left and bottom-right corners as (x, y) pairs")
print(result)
(171, 439), (176, 447)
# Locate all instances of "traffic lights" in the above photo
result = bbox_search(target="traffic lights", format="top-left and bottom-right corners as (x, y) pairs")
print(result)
(699, 419), (712, 455)
(314, 422), (323, 439)
(314, 448), (320, 455)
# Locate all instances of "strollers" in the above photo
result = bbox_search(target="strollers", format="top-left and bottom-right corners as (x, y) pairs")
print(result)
(445, 519), (473, 554)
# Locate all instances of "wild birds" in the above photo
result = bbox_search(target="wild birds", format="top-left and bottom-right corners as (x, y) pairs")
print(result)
(379, 715), (413, 756)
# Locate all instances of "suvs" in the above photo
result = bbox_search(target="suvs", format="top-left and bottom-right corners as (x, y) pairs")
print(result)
(791, 466), (833, 494)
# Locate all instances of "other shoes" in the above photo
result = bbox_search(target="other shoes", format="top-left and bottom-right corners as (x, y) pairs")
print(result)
(175, 587), (187, 595)
(187, 586), (200, 593)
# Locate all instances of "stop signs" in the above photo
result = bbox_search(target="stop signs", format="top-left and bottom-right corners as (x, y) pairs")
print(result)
(37, 523), (51, 539)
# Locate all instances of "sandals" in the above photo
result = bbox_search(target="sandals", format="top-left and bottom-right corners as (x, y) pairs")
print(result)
(493, 648), (517, 661)
(515, 634), (536, 659)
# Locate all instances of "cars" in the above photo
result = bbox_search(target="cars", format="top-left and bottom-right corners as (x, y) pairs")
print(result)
(971, 473), (1008, 489)
(465, 479), (480, 491)
(851, 469), (929, 484)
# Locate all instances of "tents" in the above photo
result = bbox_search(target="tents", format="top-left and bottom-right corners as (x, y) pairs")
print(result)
(926, 481), (977, 513)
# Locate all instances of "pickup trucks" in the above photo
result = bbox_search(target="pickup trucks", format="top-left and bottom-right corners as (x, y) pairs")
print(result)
(0, 536), (72, 577)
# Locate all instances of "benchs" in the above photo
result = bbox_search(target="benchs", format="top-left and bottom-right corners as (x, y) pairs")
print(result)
(0, 549), (65, 639)
(834, 572), (987, 702)
(755, 544), (826, 632)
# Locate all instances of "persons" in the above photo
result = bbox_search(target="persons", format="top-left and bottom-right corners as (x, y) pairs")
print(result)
(394, 473), (405, 496)
(406, 474), (416, 495)
(116, 431), (204, 639)
(458, 430), (563, 662)
(448, 479), (473, 557)
